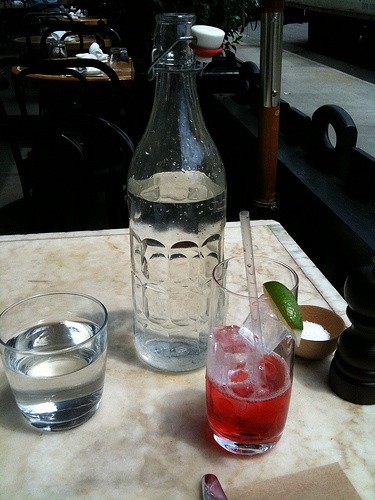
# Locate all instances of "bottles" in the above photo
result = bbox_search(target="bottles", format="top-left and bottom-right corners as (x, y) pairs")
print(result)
(326, 252), (375, 407)
(126, 12), (228, 372)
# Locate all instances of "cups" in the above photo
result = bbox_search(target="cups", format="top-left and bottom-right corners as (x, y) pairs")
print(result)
(205, 255), (300, 456)
(0, 291), (109, 433)
(109, 48), (131, 78)
(51, 41), (67, 58)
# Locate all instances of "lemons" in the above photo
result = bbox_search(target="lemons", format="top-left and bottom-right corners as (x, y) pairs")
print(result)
(263, 281), (303, 347)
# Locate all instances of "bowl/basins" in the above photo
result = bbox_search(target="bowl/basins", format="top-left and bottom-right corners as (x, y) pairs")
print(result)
(294, 304), (346, 361)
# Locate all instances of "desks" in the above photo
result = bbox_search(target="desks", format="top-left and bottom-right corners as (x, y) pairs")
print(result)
(12, 57), (135, 123)
(0, 220), (375, 500)
(12, 32), (127, 60)
(37, 14), (111, 31)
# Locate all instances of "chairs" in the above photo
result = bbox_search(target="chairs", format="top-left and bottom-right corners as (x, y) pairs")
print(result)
(0, 0), (140, 232)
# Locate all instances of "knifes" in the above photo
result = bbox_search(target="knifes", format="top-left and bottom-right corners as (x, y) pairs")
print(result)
(201, 474), (228, 500)
